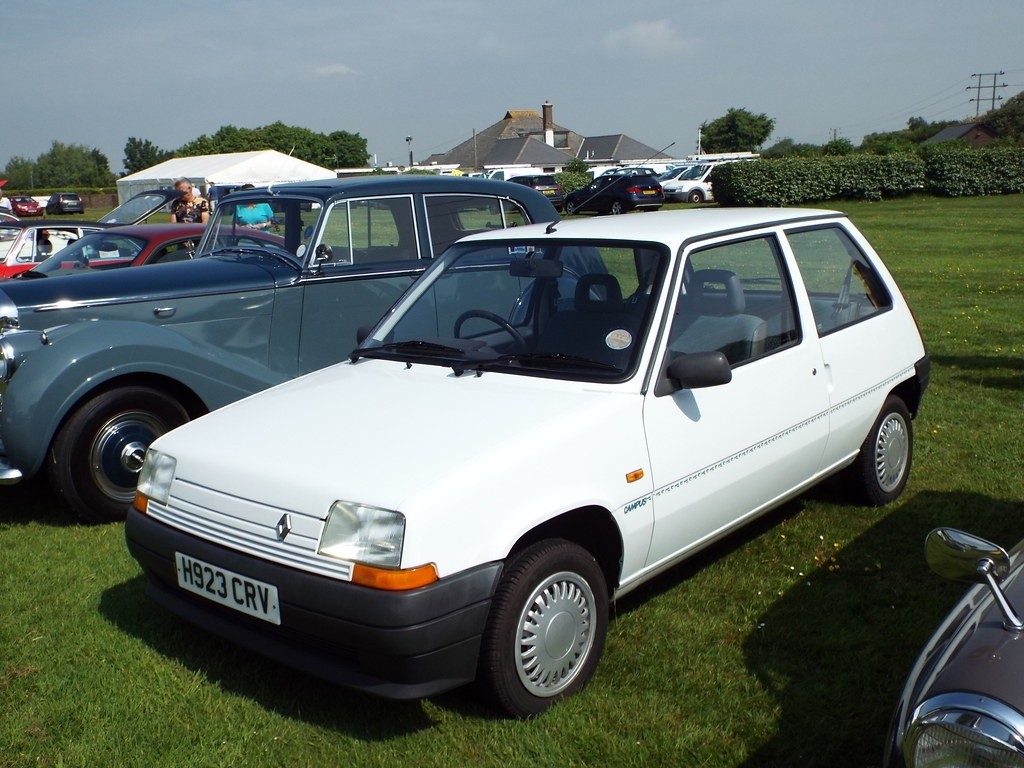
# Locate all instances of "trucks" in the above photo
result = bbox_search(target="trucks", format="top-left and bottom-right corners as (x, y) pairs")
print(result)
(479, 167), (542, 183)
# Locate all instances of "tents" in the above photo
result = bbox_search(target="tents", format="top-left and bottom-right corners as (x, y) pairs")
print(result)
(116, 150), (337, 213)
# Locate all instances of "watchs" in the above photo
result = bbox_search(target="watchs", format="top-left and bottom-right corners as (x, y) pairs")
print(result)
(264, 223), (267, 228)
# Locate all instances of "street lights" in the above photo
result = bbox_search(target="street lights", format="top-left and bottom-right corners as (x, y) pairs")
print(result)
(698, 126), (702, 155)
(406, 136), (413, 167)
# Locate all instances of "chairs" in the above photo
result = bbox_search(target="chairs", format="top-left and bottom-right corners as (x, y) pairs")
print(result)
(538, 273), (639, 367)
(667, 270), (766, 364)
(38, 239), (52, 257)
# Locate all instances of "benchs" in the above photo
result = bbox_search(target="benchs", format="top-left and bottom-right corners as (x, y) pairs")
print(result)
(680, 294), (861, 338)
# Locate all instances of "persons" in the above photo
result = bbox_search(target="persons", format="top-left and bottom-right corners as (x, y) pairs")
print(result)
(207, 182), (218, 214)
(170, 178), (211, 250)
(192, 183), (201, 197)
(0, 188), (12, 210)
(232, 183), (274, 243)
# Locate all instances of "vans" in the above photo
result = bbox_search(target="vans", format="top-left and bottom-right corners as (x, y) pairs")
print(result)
(600, 167), (660, 178)
(656, 154), (761, 203)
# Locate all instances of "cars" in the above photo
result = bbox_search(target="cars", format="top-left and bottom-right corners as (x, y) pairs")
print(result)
(123, 208), (928, 727)
(8, 196), (44, 217)
(11, 223), (287, 279)
(44, 193), (84, 215)
(0, 190), (187, 280)
(0, 207), (22, 224)
(488, 175), (564, 215)
(564, 174), (666, 216)
(0, 177), (610, 527)
